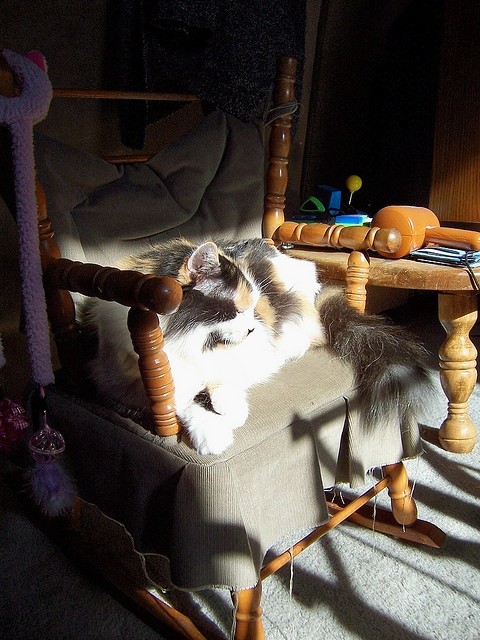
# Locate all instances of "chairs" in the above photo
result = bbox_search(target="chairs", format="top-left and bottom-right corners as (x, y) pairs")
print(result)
(0, 58), (446, 640)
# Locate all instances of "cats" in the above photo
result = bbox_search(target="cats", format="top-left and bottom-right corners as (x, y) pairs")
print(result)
(73, 236), (439, 455)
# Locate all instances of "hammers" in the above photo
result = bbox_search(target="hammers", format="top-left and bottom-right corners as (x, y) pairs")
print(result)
(370, 205), (480, 260)
